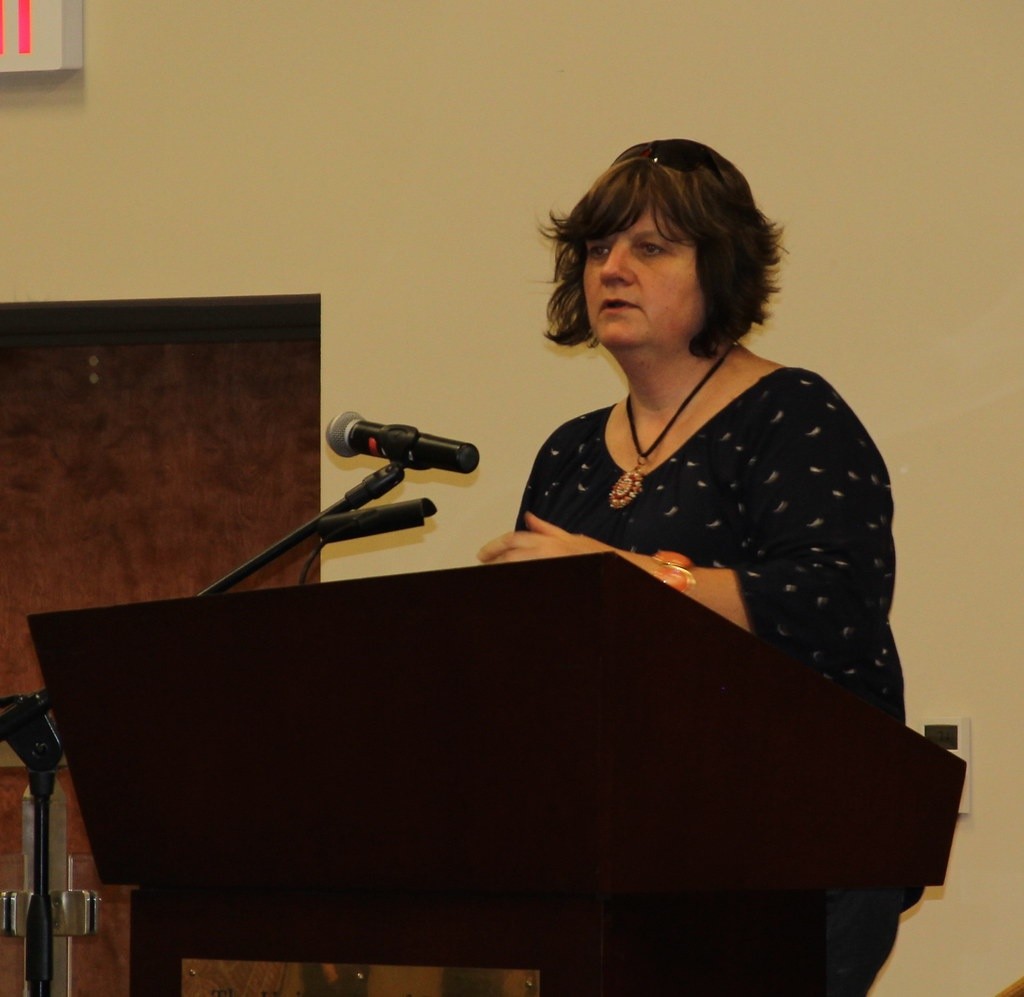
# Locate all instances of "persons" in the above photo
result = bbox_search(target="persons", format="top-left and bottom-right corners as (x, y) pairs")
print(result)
(481, 139), (906, 997)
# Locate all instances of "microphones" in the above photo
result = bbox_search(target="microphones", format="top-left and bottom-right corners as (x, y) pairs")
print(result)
(325, 411), (480, 475)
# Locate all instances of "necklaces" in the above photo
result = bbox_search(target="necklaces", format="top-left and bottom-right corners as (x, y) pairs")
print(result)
(608, 341), (738, 509)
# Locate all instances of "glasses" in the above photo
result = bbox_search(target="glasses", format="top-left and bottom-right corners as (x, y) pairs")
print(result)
(611, 138), (729, 192)
